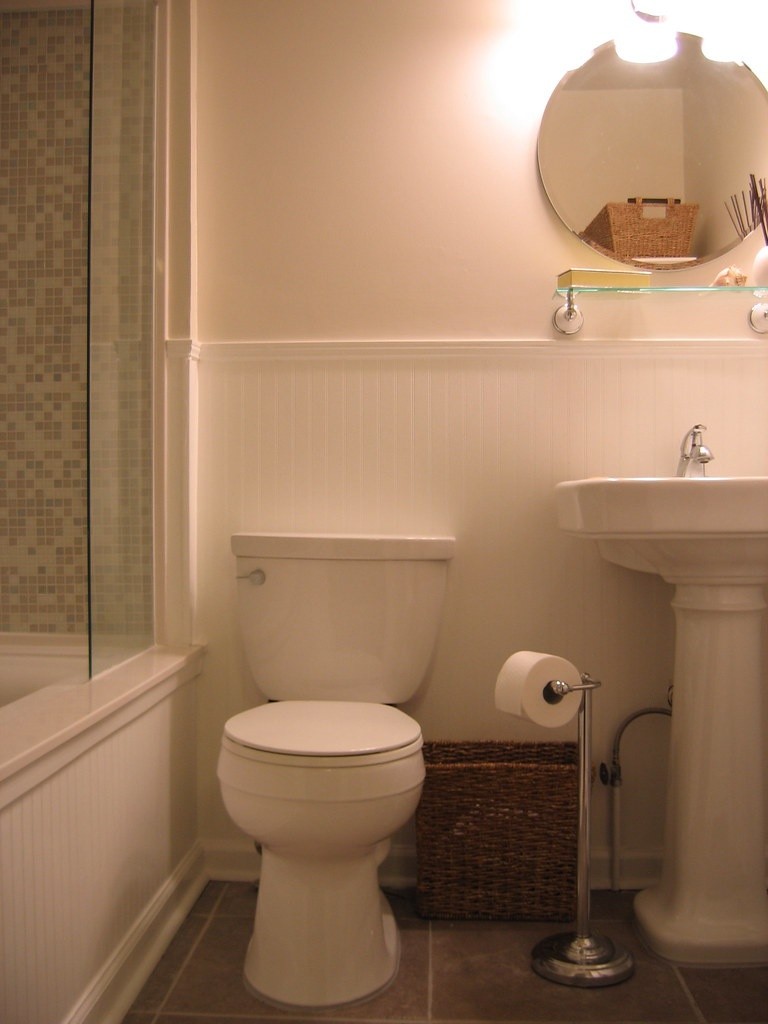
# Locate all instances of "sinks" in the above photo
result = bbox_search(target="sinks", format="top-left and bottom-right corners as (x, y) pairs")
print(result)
(556, 474), (768, 580)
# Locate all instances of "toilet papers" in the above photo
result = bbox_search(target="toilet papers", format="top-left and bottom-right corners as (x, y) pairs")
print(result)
(495, 651), (583, 728)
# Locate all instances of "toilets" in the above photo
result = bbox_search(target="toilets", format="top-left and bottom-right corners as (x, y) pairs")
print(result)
(216, 530), (461, 1015)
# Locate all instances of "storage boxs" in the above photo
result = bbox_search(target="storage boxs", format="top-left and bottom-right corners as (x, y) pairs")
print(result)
(420, 740), (584, 919)
(578, 195), (698, 269)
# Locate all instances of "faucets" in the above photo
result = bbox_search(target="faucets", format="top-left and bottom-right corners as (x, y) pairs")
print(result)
(678, 424), (713, 477)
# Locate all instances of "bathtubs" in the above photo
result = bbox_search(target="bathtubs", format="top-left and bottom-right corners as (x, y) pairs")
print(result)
(1, 628), (205, 1024)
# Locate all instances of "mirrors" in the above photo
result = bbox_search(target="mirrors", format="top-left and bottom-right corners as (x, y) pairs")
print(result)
(537, 33), (768, 269)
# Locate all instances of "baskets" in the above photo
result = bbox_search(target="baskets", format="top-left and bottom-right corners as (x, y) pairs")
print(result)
(583, 197), (701, 259)
(414, 739), (580, 924)
